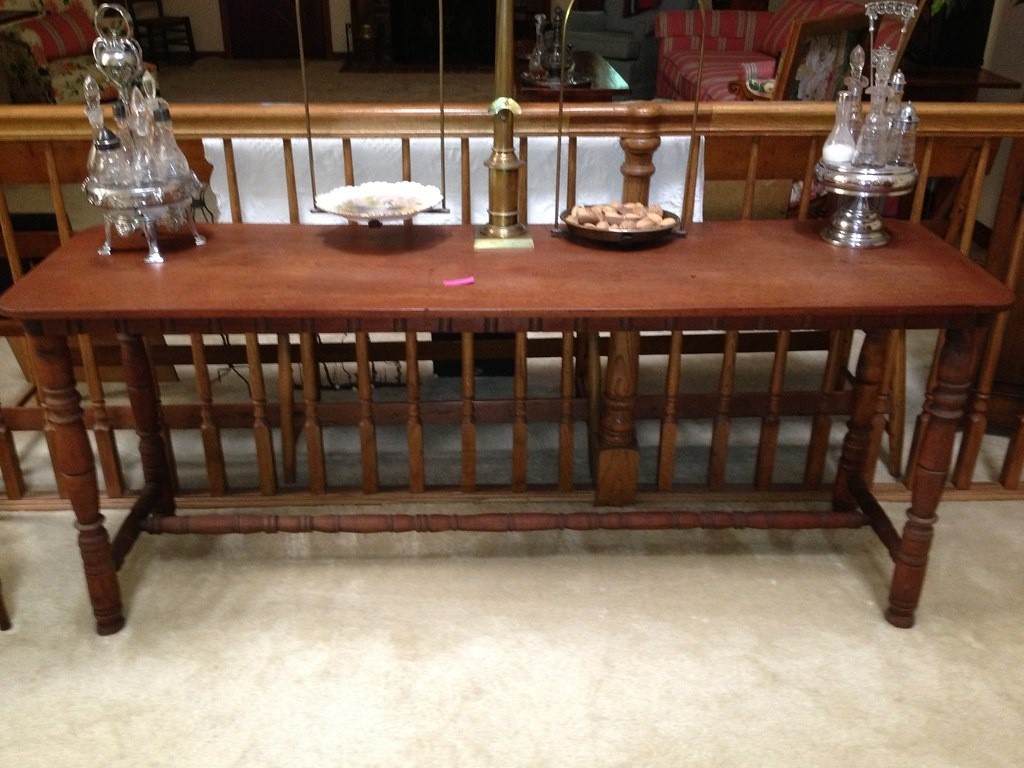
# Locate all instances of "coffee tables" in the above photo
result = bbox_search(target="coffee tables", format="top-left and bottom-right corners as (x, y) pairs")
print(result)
(514, 46), (630, 102)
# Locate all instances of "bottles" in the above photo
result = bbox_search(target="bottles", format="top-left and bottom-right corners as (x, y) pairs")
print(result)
(821, 44), (921, 172)
(529, 6), (575, 79)
(84, 71), (189, 187)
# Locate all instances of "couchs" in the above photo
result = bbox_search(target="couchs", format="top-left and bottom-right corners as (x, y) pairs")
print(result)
(565, 0), (696, 100)
(654, 0), (902, 101)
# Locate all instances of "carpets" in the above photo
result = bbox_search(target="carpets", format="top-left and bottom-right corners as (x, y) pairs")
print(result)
(339, 60), (496, 73)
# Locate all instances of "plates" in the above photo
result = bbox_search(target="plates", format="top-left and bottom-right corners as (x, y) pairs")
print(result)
(558, 206), (681, 245)
(519, 70), (590, 86)
(314, 181), (444, 221)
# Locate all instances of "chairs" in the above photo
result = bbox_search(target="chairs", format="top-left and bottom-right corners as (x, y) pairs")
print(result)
(123, 0), (197, 67)
(0, 0), (160, 105)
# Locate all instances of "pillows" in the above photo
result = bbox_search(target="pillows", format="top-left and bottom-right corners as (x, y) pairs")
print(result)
(0, 3), (99, 64)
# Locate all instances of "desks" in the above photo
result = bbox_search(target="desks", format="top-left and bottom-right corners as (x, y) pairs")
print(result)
(896, 56), (1022, 102)
(0, 218), (1020, 637)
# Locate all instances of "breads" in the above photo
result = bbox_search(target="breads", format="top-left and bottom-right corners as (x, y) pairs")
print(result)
(566, 202), (676, 230)
